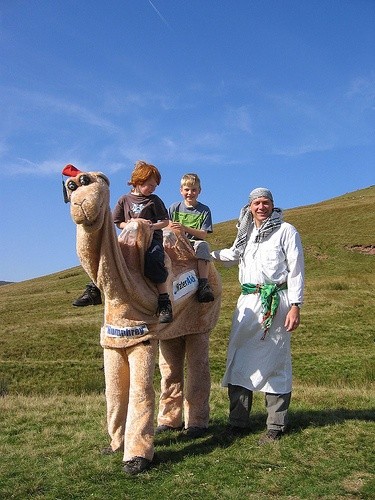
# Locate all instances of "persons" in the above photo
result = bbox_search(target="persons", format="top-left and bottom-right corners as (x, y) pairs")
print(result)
(168, 174), (214, 303)
(210, 187), (304, 447)
(72, 160), (173, 323)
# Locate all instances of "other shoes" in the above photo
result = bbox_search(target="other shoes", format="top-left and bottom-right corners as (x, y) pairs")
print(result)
(184, 425), (205, 436)
(153, 425), (173, 435)
(224, 425), (250, 436)
(259, 429), (282, 444)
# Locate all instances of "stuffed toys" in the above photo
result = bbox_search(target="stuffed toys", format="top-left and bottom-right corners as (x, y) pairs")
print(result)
(62, 164), (221, 476)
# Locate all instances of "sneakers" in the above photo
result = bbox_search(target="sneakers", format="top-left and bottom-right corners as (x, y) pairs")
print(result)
(155, 299), (173, 323)
(196, 282), (214, 302)
(122, 455), (149, 475)
(72, 284), (102, 307)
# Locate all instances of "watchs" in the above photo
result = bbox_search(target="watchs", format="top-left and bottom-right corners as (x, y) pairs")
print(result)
(291, 303), (301, 310)
